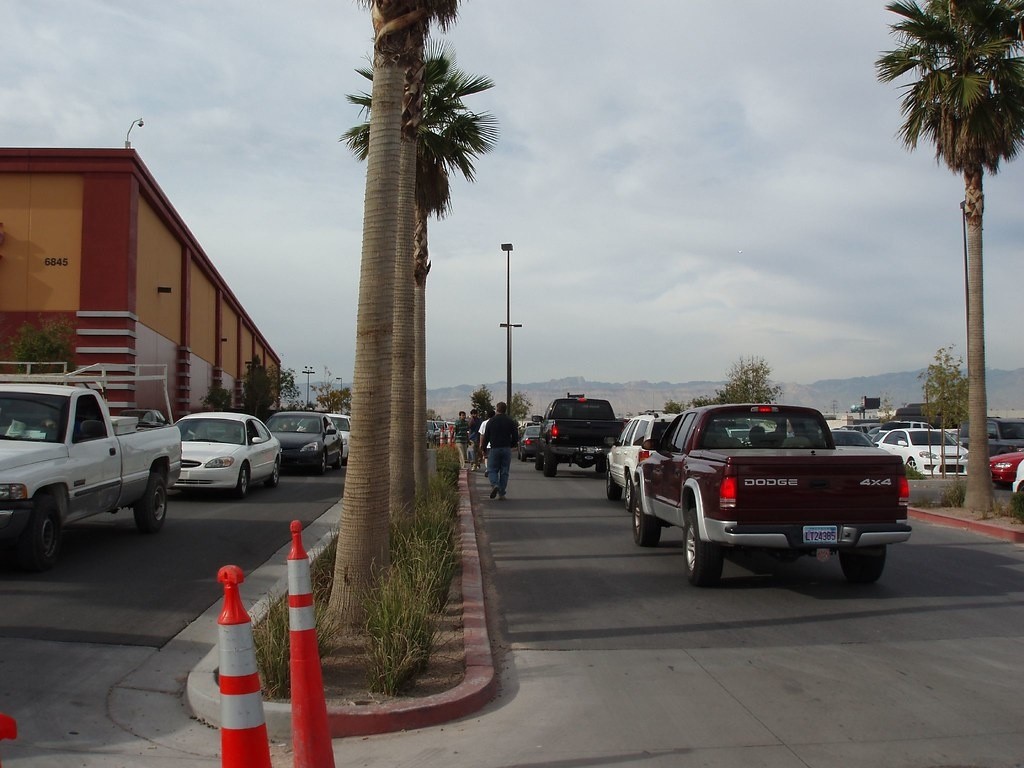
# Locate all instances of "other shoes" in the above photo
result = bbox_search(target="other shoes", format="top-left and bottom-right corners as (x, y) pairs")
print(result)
(499, 496), (506, 500)
(490, 485), (498, 499)
(472, 463), (476, 471)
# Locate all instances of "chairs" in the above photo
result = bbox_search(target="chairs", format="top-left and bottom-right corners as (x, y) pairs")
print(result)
(782, 436), (811, 448)
(278, 419), (290, 431)
(223, 424), (239, 442)
(710, 437), (740, 448)
(306, 421), (319, 433)
(184, 424), (207, 440)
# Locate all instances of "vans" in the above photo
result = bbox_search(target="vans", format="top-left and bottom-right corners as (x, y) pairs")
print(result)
(956, 415), (1024, 457)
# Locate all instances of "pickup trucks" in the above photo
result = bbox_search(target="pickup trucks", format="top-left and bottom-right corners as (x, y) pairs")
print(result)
(0, 360), (183, 573)
(627, 402), (915, 584)
(531, 392), (626, 477)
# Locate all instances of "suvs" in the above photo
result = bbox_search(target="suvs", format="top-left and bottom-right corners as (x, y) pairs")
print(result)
(604, 409), (680, 501)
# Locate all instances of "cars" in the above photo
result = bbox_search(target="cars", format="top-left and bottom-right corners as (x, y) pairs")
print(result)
(261, 410), (344, 473)
(874, 428), (969, 478)
(1011, 459), (1024, 492)
(829, 417), (936, 455)
(324, 412), (351, 464)
(166, 412), (283, 496)
(427, 419), (455, 449)
(517, 425), (541, 463)
(987, 446), (1024, 486)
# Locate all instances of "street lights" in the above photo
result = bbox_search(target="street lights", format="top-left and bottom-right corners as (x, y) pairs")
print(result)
(500, 239), (517, 413)
(336, 376), (343, 397)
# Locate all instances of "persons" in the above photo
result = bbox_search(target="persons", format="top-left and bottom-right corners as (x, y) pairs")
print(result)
(451, 411), (469, 468)
(715, 422), (728, 441)
(39, 401), (81, 435)
(222, 422), (241, 437)
(749, 426), (765, 447)
(280, 419), (298, 432)
(193, 424), (211, 439)
(478, 410), (495, 477)
(481, 402), (518, 499)
(468, 409), (482, 472)
(306, 421), (332, 433)
